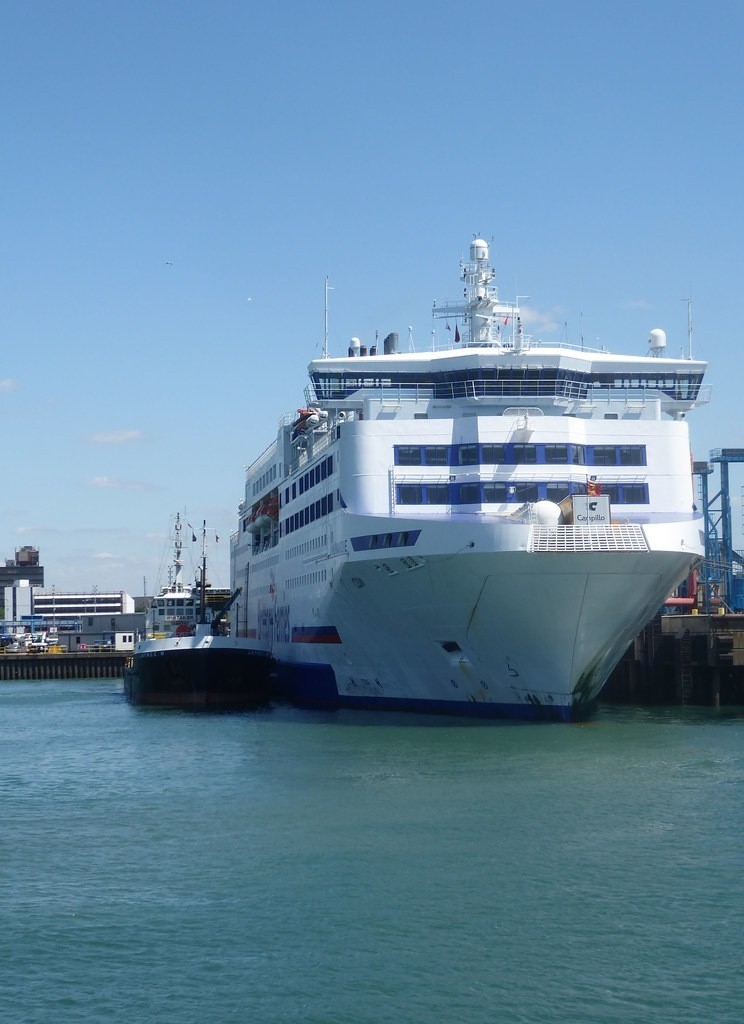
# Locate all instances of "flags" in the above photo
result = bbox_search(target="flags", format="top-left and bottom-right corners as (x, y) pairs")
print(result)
(585, 482), (602, 497)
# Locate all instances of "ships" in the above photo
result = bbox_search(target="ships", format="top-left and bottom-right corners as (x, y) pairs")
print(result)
(226, 230), (712, 724)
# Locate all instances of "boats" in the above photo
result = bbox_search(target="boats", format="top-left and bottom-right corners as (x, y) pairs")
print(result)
(244, 513), (255, 533)
(122, 505), (273, 711)
(267, 498), (277, 519)
(255, 505), (271, 526)
